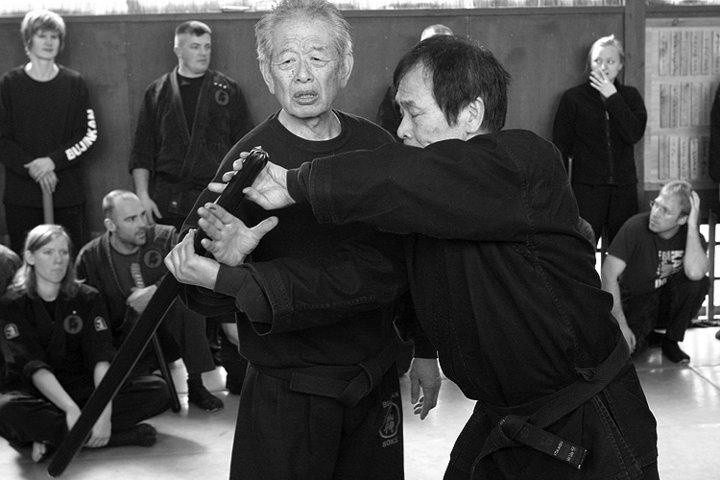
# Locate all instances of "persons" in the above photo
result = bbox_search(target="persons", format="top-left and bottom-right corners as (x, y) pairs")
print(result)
(163, 36), (662, 479)
(599, 179), (711, 366)
(71, 189), (225, 413)
(0, 8), (101, 256)
(219, 321), (252, 393)
(127, 20), (257, 236)
(173, 0), (442, 480)
(0, 222), (171, 465)
(552, 33), (647, 277)
(375, 24), (454, 144)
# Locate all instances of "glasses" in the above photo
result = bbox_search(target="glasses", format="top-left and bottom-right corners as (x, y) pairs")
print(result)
(649, 200), (677, 216)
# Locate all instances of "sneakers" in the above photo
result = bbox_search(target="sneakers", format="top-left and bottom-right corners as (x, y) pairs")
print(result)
(32, 438), (63, 463)
(187, 378), (224, 412)
(110, 424), (158, 447)
(662, 336), (689, 364)
(227, 373), (244, 394)
